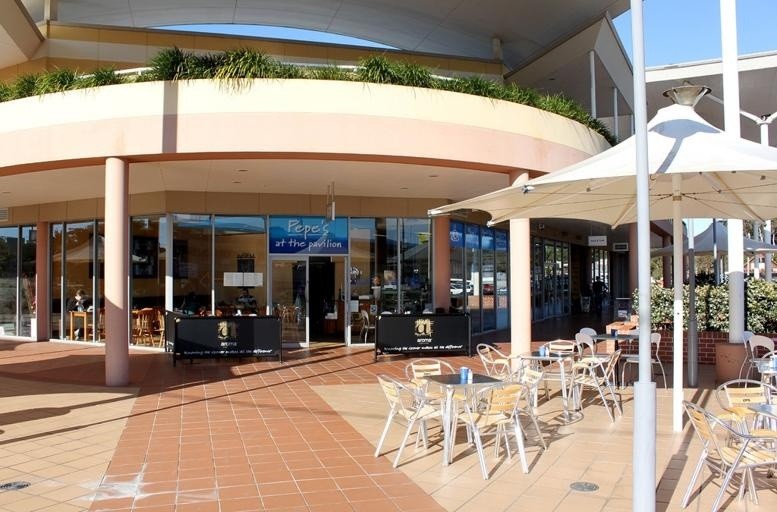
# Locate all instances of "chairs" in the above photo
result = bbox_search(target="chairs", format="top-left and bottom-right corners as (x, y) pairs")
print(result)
(70, 308), (165, 348)
(359, 310), (375, 343)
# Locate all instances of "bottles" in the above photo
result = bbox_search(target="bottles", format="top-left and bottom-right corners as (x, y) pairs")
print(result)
(768, 355), (776, 371)
(544, 347), (549, 356)
(467, 368), (473, 385)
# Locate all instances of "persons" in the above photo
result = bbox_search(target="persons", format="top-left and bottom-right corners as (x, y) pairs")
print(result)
(593, 275), (608, 307)
(181, 291), (201, 312)
(66, 289), (91, 342)
(235, 288), (256, 310)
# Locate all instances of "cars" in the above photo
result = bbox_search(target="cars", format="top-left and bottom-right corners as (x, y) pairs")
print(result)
(449, 266), (507, 296)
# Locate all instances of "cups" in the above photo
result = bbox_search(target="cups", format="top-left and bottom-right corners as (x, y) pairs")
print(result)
(460, 367), (468, 384)
(539, 346), (545, 356)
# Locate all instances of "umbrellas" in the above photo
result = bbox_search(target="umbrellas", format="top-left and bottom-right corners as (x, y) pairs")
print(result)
(427, 99), (777, 433)
